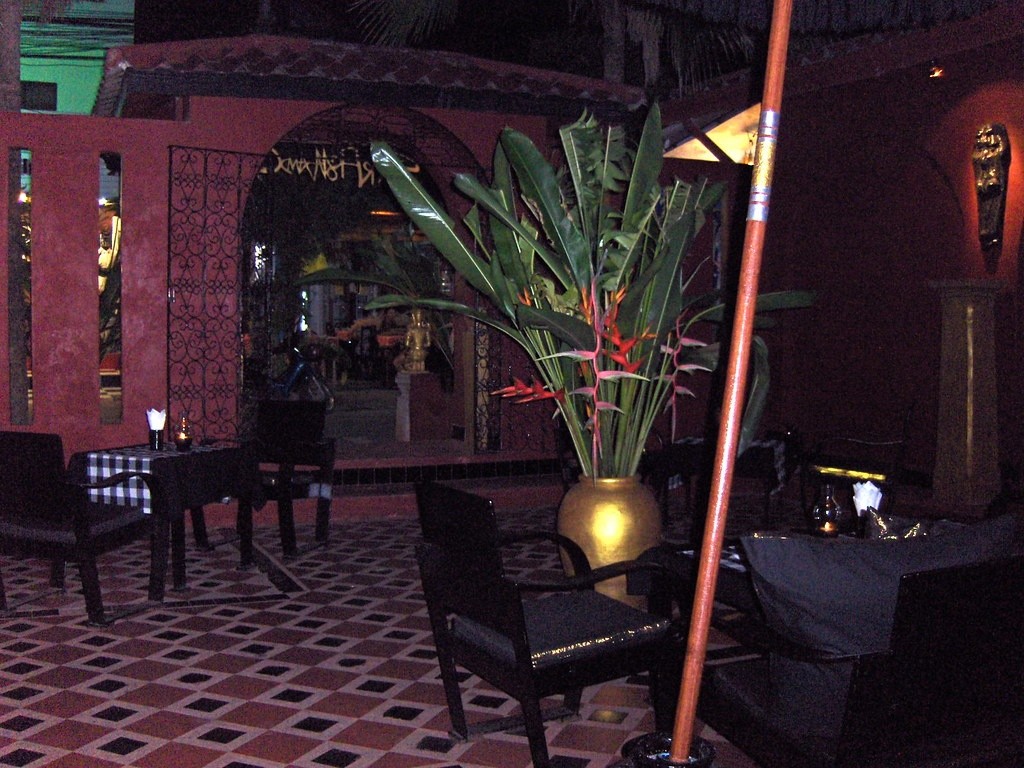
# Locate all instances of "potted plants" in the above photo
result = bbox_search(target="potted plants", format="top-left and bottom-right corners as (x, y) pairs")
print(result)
(276, 98), (819, 613)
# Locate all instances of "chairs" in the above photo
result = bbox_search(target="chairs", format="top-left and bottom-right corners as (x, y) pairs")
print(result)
(0, 430), (170, 626)
(235, 396), (335, 558)
(554, 400), (917, 532)
(413, 477), (693, 768)
(668, 557), (1024, 768)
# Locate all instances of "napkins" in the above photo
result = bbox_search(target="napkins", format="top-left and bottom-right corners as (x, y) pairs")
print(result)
(852, 479), (882, 517)
(145, 408), (167, 430)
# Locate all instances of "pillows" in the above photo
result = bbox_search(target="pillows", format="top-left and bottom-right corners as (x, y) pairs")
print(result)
(740, 511), (1024, 740)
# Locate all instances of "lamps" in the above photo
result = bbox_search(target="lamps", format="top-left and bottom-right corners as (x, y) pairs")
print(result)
(929, 59), (943, 78)
(663, 98), (761, 166)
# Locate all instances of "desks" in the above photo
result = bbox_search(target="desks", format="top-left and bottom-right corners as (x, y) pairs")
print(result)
(626, 525), (866, 621)
(69, 440), (260, 593)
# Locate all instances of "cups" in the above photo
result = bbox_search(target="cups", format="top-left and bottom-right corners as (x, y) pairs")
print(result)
(149, 430), (164, 451)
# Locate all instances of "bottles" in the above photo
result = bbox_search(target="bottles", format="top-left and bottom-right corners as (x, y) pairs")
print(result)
(174, 417), (193, 450)
(814, 483), (841, 534)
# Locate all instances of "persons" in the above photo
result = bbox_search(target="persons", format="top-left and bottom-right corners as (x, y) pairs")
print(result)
(972, 124), (1006, 249)
(393, 308), (433, 373)
